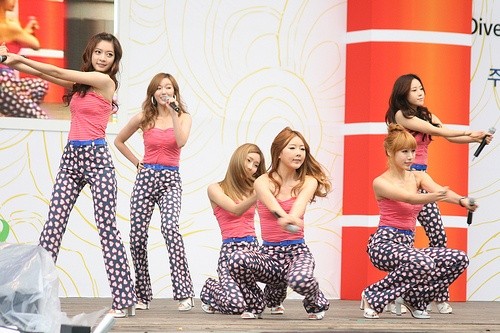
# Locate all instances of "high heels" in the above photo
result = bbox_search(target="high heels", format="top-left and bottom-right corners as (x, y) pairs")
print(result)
(307, 310), (326, 320)
(384, 302), (407, 315)
(359, 290), (380, 319)
(177, 297), (195, 311)
(426, 301), (452, 314)
(395, 296), (431, 319)
(108, 304), (136, 318)
(241, 312), (264, 319)
(135, 301), (150, 310)
(201, 301), (214, 314)
(268, 301), (284, 315)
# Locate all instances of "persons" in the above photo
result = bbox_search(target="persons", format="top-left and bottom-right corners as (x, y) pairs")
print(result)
(200, 142), (267, 315)
(115, 72), (195, 312)
(0, 0), (48, 119)
(360, 123), (478, 319)
(0, 32), (137, 318)
(385, 72), (494, 315)
(226, 126), (336, 321)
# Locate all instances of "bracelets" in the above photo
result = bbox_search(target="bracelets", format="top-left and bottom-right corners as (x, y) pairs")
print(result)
(137, 163), (143, 169)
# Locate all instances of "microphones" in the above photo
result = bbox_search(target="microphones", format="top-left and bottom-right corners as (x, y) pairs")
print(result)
(165, 95), (180, 111)
(467, 198), (476, 225)
(273, 210), (297, 232)
(474, 127), (496, 157)
(0, 54), (26, 63)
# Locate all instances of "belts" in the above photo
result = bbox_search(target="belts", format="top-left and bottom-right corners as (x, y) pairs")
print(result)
(411, 164), (428, 171)
(262, 239), (306, 247)
(222, 235), (256, 243)
(69, 138), (108, 148)
(379, 226), (412, 235)
(141, 163), (179, 172)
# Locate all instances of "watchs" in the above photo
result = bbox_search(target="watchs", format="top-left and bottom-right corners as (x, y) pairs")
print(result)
(459, 196), (466, 207)
